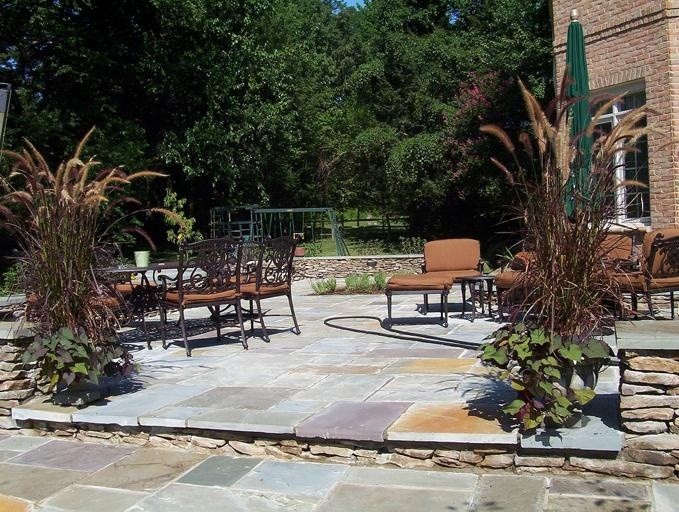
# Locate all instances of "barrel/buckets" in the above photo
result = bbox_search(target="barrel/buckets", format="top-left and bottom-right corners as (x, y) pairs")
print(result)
(134, 251), (150, 267)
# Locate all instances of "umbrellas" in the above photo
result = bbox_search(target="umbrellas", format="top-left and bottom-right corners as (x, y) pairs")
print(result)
(558, 8), (603, 238)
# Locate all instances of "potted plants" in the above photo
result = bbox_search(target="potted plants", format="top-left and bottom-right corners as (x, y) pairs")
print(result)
(475, 63), (679, 432)
(0, 125), (188, 408)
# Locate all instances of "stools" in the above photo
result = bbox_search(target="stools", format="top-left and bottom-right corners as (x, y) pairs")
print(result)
(384, 274), (453, 327)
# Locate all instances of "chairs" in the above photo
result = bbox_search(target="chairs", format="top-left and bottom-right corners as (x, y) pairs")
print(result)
(23, 236), (301, 358)
(422, 229), (679, 324)
